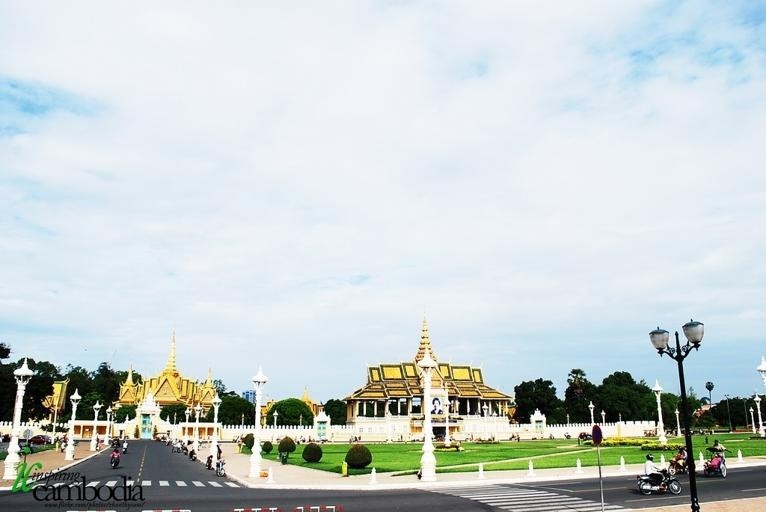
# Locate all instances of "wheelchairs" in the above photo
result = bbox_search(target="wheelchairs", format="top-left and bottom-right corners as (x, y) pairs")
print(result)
(703, 448), (728, 477)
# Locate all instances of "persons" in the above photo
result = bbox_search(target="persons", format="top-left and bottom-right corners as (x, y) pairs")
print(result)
(431, 398), (443, 414)
(60, 432), (223, 470)
(644, 428), (725, 491)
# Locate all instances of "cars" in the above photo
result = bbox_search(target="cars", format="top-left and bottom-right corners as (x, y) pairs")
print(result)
(0, 433), (64, 451)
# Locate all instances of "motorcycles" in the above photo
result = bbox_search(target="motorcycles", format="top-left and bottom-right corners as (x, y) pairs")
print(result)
(666, 455), (690, 476)
(108, 439), (129, 468)
(639, 466), (683, 495)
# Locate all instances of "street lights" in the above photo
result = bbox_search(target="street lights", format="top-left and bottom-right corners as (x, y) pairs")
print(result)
(707, 356), (766, 438)
(565, 400), (623, 440)
(0, 356), (34, 480)
(653, 378), (667, 445)
(161, 365), (278, 482)
(104, 405), (113, 446)
(63, 386), (82, 462)
(673, 407), (684, 436)
(417, 342), (500, 482)
(650, 319), (706, 511)
(89, 399), (102, 452)
